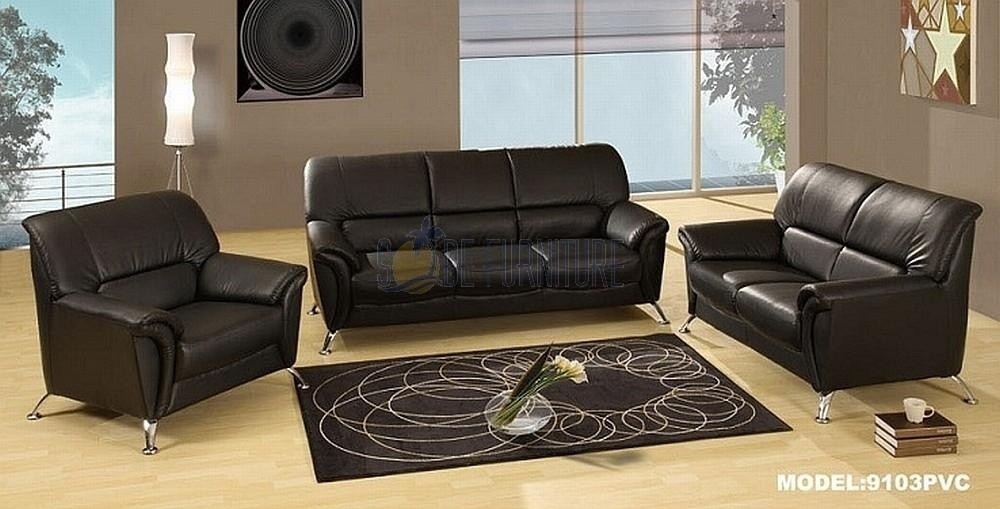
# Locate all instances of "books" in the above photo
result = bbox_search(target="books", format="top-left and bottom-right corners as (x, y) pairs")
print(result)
(872, 410), (958, 458)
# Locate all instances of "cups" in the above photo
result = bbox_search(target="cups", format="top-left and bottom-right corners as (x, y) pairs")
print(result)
(903, 397), (935, 424)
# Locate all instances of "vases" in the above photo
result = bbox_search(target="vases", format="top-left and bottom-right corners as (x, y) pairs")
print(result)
(483, 389), (551, 437)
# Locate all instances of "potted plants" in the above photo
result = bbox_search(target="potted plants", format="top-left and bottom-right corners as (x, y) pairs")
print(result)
(756, 98), (791, 194)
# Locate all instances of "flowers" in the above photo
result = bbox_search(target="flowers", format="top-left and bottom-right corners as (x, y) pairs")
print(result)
(487, 353), (591, 433)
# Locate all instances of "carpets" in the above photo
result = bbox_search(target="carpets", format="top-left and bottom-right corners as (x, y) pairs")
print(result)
(289, 332), (798, 484)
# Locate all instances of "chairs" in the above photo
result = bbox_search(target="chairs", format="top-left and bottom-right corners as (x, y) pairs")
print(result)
(18, 188), (310, 458)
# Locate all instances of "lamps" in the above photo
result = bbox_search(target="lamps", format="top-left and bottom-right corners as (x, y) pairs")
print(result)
(160, 31), (199, 202)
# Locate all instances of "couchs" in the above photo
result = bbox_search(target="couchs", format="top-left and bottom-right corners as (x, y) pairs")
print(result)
(675, 158), (984, 426)
(300, 140), (675, 358)
